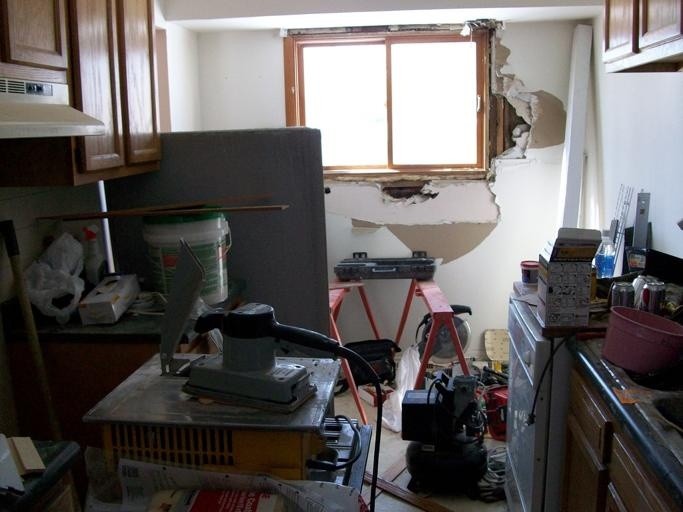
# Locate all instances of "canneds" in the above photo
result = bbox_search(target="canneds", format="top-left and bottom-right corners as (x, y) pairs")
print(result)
(612, 282), (634, 307)
(641, 282), (665, 314)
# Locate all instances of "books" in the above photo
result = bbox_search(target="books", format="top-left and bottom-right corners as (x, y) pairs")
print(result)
(148, 489), (285, 512)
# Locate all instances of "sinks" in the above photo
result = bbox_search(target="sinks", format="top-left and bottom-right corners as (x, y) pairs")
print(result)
(648, 393), (682, 434)
(623, 367), (683, 391)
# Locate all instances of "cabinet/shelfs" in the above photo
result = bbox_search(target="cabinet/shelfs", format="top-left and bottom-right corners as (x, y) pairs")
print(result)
(2, 0), (74, 71)
(0, 1), (162, 189)
(601, 0), (683, 74)
(560, 368), (683, 512)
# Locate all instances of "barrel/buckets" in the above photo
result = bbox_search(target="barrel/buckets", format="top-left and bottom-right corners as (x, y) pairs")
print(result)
(141, 204), (233, 306)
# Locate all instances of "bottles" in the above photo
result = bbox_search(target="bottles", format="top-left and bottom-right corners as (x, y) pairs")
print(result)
(595, 237), (616, 279)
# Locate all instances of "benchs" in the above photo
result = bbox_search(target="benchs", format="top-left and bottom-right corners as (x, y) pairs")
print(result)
(329, 279), (380, 425)
(391, 277), (471, 377)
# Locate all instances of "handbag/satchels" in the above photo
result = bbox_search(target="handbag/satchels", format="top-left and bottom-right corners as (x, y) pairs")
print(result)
(343, 339), (395, 385)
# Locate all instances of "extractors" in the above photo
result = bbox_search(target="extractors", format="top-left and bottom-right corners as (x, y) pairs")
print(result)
(1, 77), (106, 141)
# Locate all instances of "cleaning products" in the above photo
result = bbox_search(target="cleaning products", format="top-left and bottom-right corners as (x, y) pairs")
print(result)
(81, 223), (106, 285)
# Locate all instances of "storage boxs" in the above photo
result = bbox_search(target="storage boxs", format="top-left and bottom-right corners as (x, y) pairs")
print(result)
(536, 227), (602, 330)
